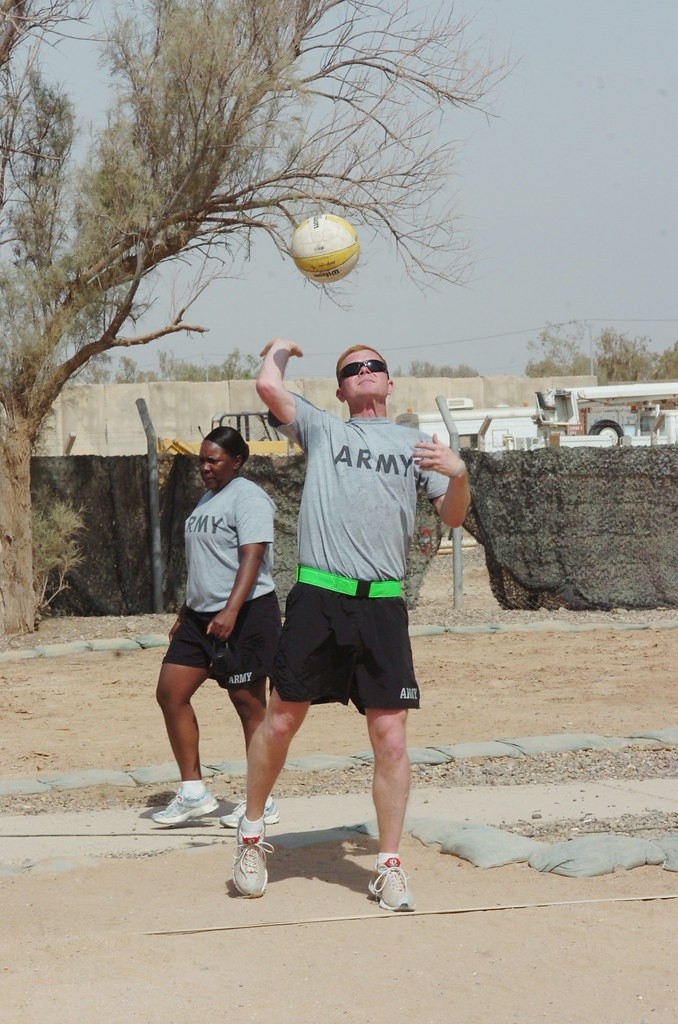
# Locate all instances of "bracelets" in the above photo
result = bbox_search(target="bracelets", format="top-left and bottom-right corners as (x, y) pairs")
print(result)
(450, 461), (464, 478)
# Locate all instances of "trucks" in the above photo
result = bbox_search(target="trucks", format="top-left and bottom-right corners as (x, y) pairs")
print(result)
(395, 397), (545, 450)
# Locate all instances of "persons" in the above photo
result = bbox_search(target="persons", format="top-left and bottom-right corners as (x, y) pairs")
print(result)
(231, 341), (470, 910)
(152, 426), (282, 827)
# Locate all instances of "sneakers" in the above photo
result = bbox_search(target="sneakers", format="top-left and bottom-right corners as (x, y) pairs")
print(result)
(151, 788), (219, 825)
(232, 815), (274, 898)
(368, 862), (416, 911)
(219, 795), (279, 829)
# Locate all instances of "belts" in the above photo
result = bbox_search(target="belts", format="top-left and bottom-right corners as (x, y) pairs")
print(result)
(295, 564), (402, 598)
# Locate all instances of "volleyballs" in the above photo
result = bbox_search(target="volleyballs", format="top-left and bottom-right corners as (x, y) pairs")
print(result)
(290, 212), (360, 285)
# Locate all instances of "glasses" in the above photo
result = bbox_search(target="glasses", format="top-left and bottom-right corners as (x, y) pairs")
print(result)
(337, 359), (389, 388)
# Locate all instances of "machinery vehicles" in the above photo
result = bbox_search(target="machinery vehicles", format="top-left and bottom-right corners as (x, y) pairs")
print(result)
(158, 411), (304, 488)
